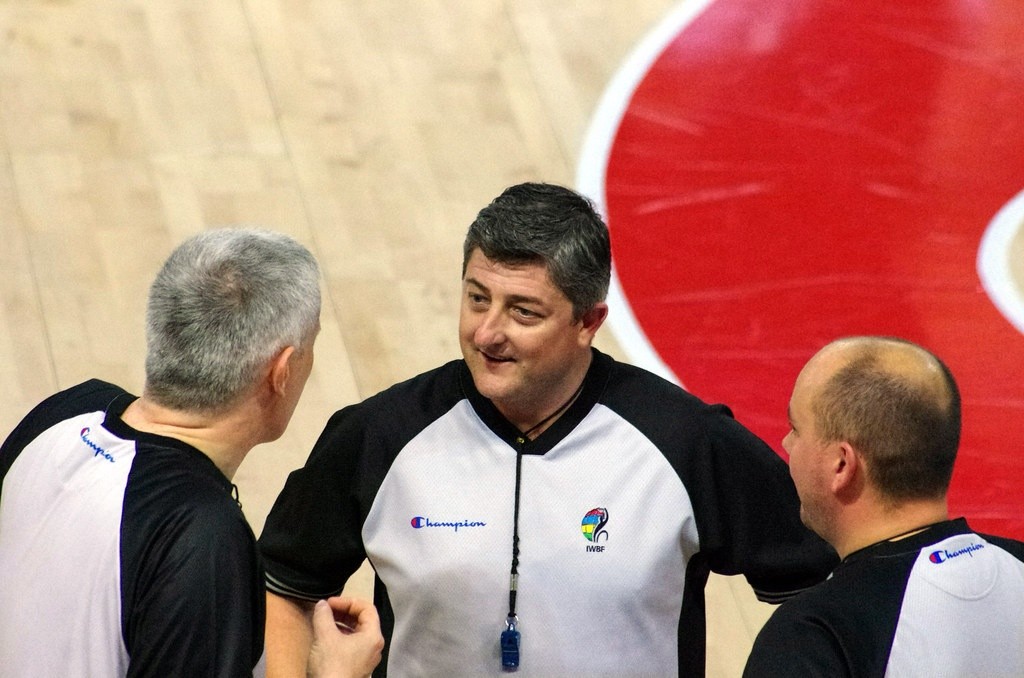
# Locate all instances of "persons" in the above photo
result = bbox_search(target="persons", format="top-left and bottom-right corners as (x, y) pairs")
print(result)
(742, 337), (1024, 678)
(258, 182), (839, 678)
(0, 226), (384, 678)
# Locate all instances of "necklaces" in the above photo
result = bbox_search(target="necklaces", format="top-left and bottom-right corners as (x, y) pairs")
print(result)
(501, 358), (594, 668)
(842, 520), (950, 565)
